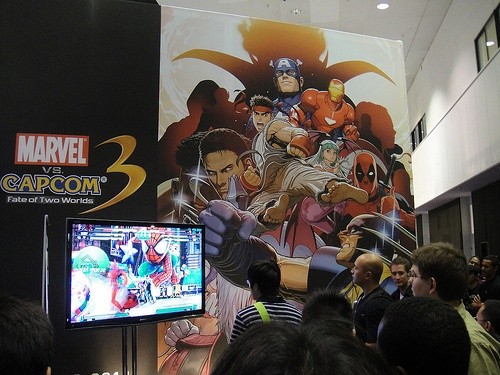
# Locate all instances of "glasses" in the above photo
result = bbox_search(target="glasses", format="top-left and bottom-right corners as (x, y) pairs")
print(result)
(246, 279), (252, 284)
(474, 317), (485, 322)
(407, 272), (424, 280)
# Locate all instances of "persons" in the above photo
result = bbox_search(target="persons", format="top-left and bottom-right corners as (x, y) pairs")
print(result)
(229, 260), (302, 343)
(301, 290), (357, 338)
(391, 256), (414, 301)
(0, 295), (56, 375)
(209, 320), (388, 375)
(351, 252), (390, 352)
(376, 296), (472, 375)
(463, 255), (500, 342)
(408, 241), (500, 375)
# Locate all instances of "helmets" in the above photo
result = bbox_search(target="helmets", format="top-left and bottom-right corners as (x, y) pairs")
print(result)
(72, 245), (111, 276)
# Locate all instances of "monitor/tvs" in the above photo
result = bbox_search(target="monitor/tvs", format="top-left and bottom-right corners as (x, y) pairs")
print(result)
(64, 218), (205, 330)
